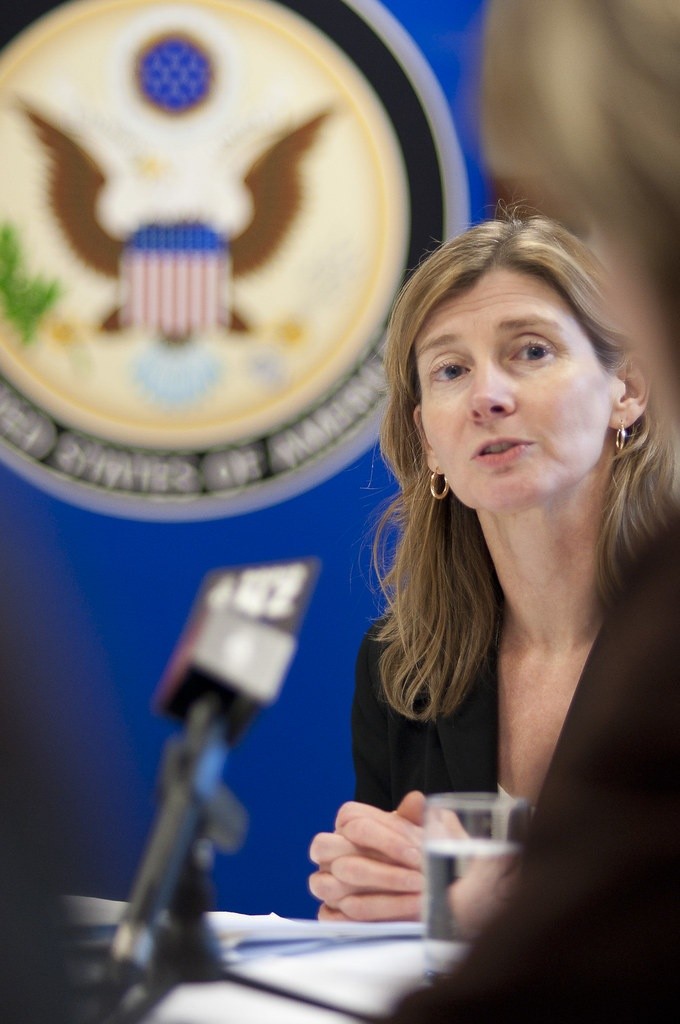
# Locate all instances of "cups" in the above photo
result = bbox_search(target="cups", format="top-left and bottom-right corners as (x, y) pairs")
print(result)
(423, 791), (535, 964)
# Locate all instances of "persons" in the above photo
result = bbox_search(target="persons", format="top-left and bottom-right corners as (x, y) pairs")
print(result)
(379, 1), (680, 1024)
(308, 218), (680, 934)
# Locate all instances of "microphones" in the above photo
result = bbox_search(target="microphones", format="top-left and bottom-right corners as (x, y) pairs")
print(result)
(104, 555), (325, 990)
